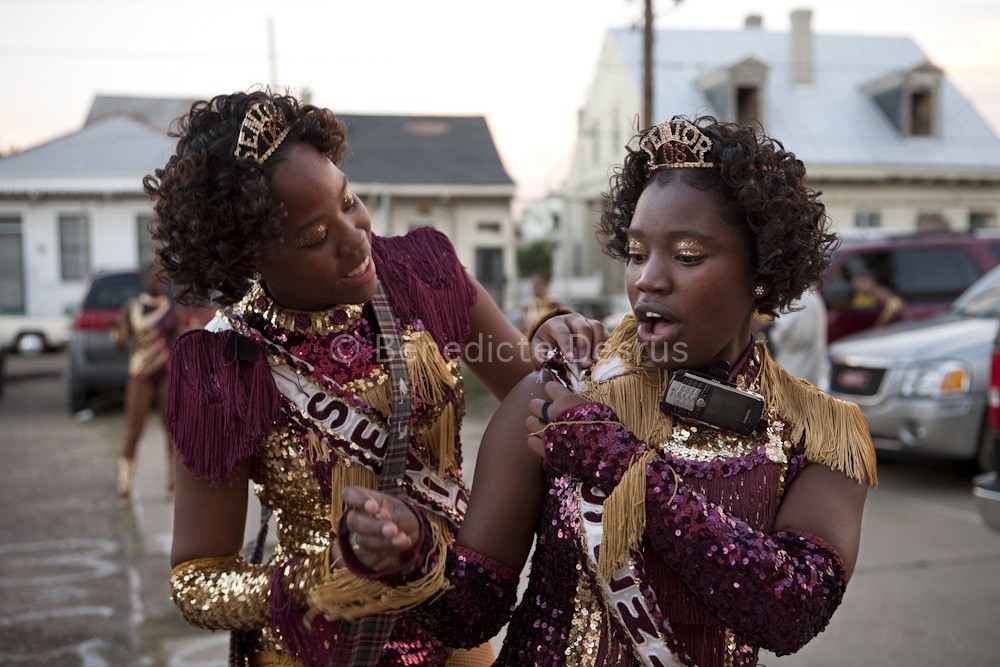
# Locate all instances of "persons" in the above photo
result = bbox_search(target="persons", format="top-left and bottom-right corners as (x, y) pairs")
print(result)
(112, 261), (187, 499)
(768, 284), (830, 392)
(143, 92), (610, 667)
(851, 266), (903, 324)
(336, 116), (879, 667)
(531, 270), (552, 309)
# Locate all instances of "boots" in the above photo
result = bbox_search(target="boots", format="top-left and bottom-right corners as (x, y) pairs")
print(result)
(120, 453), (136, 495)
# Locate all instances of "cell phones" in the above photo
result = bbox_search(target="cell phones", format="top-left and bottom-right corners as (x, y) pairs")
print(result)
(659, 369), (765, 439)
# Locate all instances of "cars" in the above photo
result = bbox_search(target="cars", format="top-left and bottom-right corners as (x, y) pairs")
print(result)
(67, 269), (177, 417)
(1, 314), (72, 355)
(828, 261), (999, 465)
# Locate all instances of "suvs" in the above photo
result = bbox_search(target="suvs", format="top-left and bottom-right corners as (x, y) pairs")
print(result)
(811, 235), (999, 346)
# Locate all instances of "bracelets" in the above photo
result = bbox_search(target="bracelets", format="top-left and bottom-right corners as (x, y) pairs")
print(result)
(542, 401), (552, 420)
(527, 307), (572, 345)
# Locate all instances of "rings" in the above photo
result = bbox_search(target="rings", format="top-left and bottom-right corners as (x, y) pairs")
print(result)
(352, 532), (360, 549)
